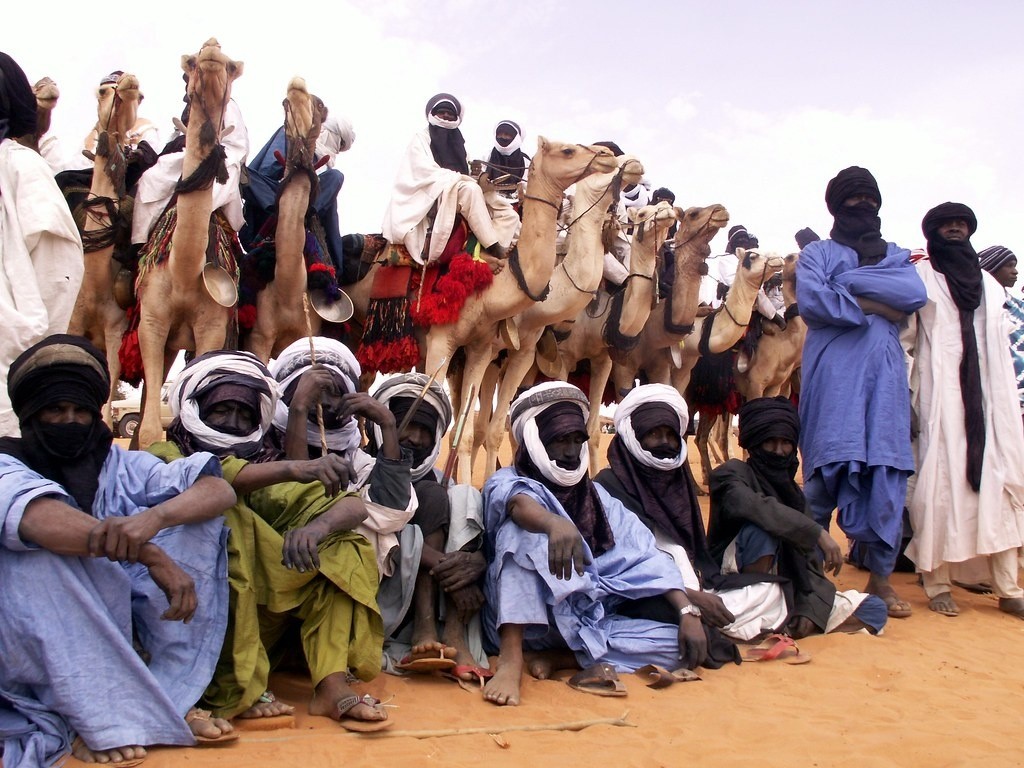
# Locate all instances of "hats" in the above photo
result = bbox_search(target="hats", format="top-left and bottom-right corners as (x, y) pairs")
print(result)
(496, 119), (521, 140)
(975, 245), (1017, 274)
(426, 92), (461, 121)
(825, 165), (881, 216)
(2, 53), (36, 139)
(922, 200), (978, 238)
(7, 333), (800, 452)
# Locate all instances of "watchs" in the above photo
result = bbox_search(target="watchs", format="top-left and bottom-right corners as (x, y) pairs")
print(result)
(679, 605), (702, 618)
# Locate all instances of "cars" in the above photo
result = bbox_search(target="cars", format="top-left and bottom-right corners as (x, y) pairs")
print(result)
(110, 380), (177, 438)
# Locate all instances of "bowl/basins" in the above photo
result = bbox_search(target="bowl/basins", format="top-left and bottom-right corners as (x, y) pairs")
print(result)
(536, 330), (561, 378)
(737, 347), (757, 373)
(501, 317), (521, 351)
(114, 264), (136, 309)
(310, 288), (354, 322)
(202, 263), (237, 308)
(666, 345), (682, 370)
(608, 345), (628, 366)
(792, 370), (801, 394)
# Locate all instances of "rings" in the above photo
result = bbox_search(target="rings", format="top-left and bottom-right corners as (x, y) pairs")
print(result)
(830, 561), (832, 564)
(476, 167), (478, 171)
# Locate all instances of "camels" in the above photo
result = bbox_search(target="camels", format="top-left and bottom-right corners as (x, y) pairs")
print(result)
(30, 36), (335, 454)
(336, 135), (808, 490)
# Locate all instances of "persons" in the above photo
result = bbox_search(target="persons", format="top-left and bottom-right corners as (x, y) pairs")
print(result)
(381, 91), (522, 275)
(590, 140), (678, 296)
(483, 119), (571, 240)
(592, 383), (798, 641)
(706, 394), (889, 636)
(83, 69), (160, 182)
(137, 336), (422, 734)
(352, 369), (493, 681)
(248, 116), (356, 276)
(480, 381), (709, 706)
(716, 222), (789, 329)
(130, 72), (250, 245)
(0, 334), (238, 768)
(796, 166), (928, 618)
(895, 200), (1024, 620)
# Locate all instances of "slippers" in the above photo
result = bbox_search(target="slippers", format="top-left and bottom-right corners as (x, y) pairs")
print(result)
(329, 689), (394, 733)
(632, 662), (702, 690)
(455, 662), (495, 694)
(394, 637), (458, 674)
(184, 708), (241, 743)
(70, 735), (149, 768)
(230, 689), (295, 726)
(741, 635), (812, 665)
(929, 598), (959, 617)
(1006, 609), (1024, 620)
(864, 590), (913, 618)
(565, 661), (628, 697)
